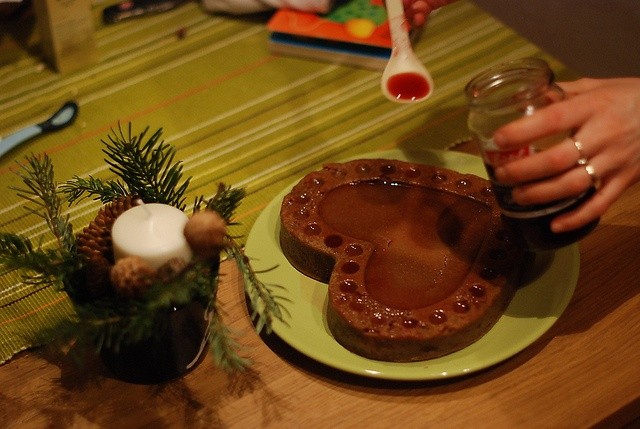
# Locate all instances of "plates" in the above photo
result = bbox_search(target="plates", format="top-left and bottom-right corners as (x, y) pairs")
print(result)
(244, 146), (581, 383)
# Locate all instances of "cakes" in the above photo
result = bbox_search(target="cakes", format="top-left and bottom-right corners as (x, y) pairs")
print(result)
(279, 158), (518, 364)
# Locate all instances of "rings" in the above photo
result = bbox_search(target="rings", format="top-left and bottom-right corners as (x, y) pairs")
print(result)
(577, 158), (602, 190)
(569, 133), (588, 165)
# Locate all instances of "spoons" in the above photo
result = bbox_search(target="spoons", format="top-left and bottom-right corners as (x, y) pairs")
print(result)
(381, 0), (433, 104)
(0, 101), (79, 161)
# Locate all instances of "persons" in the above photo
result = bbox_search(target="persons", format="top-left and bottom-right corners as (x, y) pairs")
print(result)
(492, 75), (640, 234)
